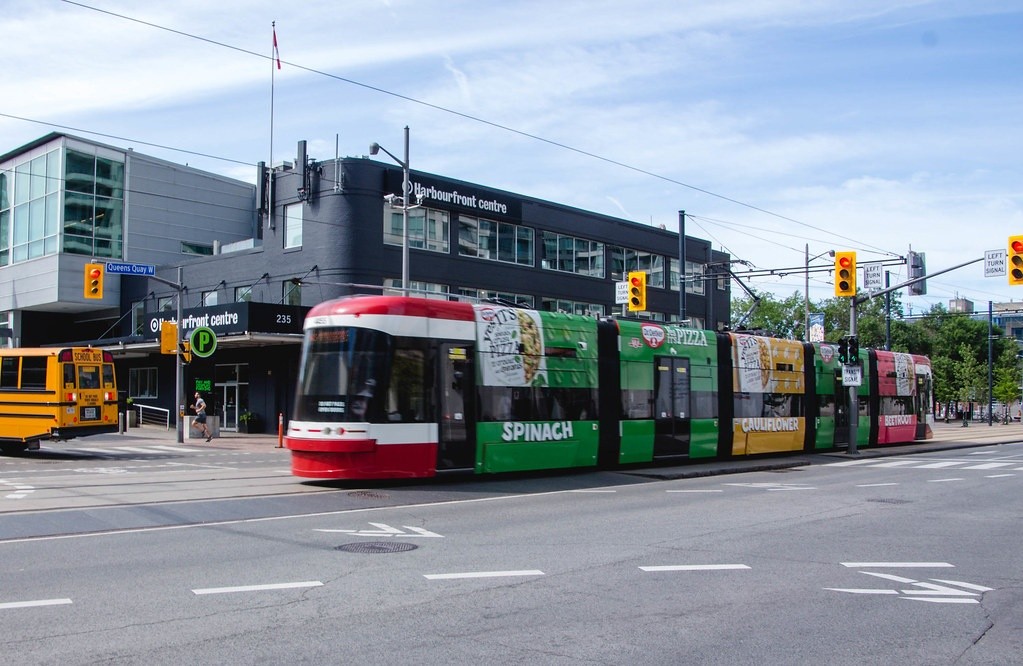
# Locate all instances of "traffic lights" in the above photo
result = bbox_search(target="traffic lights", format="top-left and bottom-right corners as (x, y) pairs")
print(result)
(1008, 235), (1023, 285)
(838, 338), (848, 363)
(629, 271), (647, 311)
(836, 251), (856, 297)
(179, 353), (192, 362)
(849, 339), (859, 364)
(179, 343), (192, 352)
(85, 264), (103, 299)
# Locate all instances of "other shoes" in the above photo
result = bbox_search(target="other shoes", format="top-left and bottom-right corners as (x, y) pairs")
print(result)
(202, 431), (205, 438)
(206, 436), (212, 442)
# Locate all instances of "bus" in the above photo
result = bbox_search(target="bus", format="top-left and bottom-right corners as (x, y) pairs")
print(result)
(0, 346), (119, 457)
(287, 294), (936, 483)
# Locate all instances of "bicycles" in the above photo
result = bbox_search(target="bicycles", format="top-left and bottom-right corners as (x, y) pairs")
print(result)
(978, 412), (1012, 425)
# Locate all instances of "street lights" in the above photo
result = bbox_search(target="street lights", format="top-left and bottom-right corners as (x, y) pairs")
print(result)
(370, 125), (410, 298)
(805, 243), (835, 342)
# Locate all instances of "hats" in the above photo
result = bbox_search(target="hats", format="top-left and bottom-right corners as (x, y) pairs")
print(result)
(364, 378), (376, 386)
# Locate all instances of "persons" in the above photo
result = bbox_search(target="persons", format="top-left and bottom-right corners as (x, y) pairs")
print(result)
(190, 391), (213, 442)
(356, 378), (377, 398)
